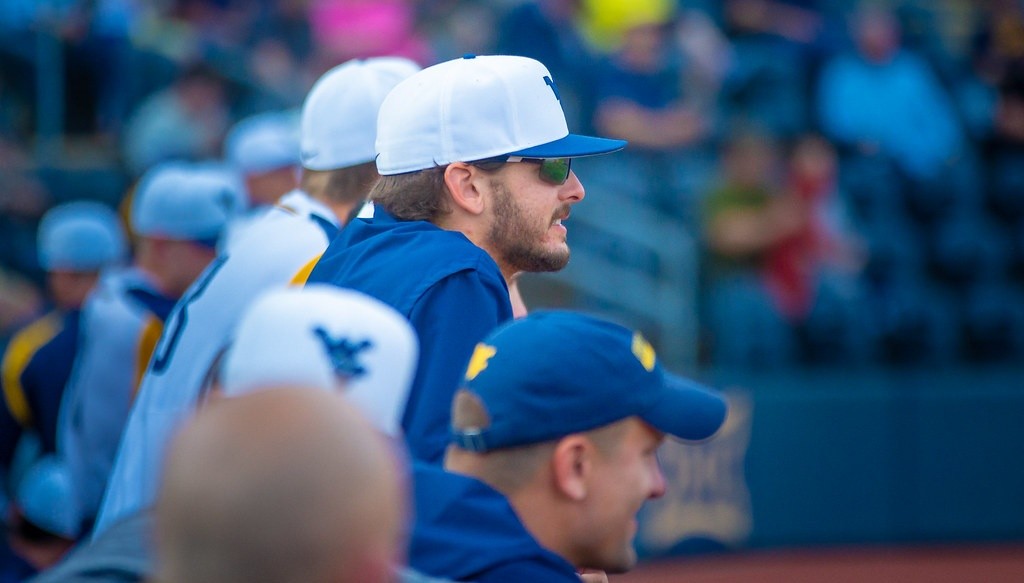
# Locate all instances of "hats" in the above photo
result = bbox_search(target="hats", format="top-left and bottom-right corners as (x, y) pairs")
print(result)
(449, 310), (732, 453)
(131, 166), (242, 240)
(298, 58), (421, 172)
(375, 55), (627, 176)
(39, 206), (123, 272)
(220, 282), (420, 439)
(224, 107), (301, 176)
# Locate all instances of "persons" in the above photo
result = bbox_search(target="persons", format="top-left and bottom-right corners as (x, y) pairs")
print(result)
(600, 0), (1021, 356)
(401, 304), (698, 583)
(0, 458), (92, 579)
(4, 189), (129, 438)
(263, 54), (597, 465)
(143, 377), (406, 583)
(120, 50), (225, 185)
(39, 166), (253, 522)
(223, 107), (310, 226)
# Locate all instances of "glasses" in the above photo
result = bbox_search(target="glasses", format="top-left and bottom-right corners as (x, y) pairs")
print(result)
(520, 158), (573, 185)
